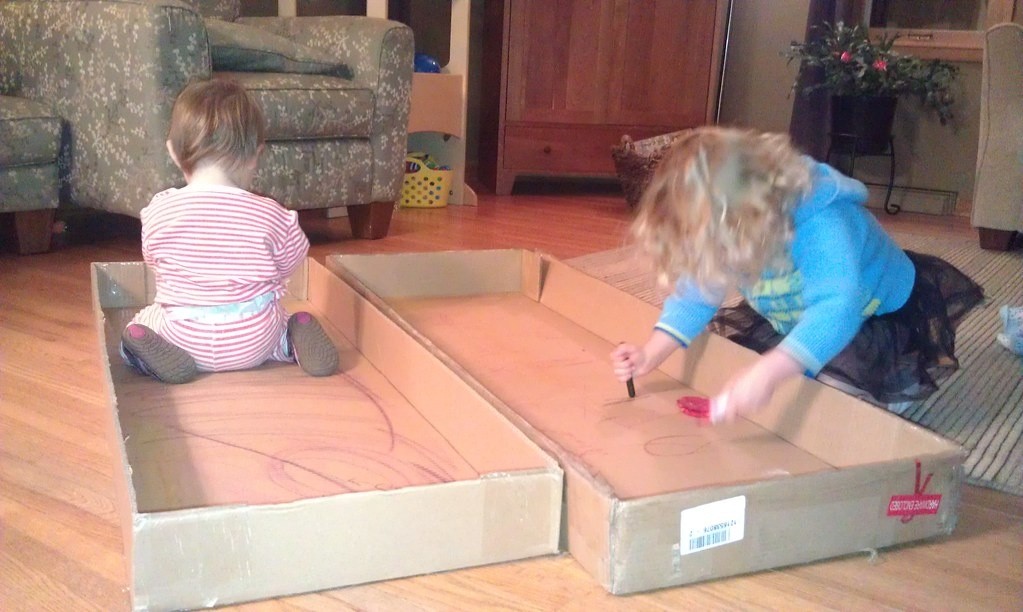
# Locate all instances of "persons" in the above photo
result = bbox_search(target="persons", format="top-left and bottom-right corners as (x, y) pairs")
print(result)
(611, 128), (985, 425)
(118, 79), (339, 384)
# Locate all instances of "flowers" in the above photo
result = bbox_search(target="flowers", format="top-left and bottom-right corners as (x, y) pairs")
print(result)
(775, 18), (963, 128)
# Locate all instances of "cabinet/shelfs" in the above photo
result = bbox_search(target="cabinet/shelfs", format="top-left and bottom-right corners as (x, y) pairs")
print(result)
(497, 0), (727, 195)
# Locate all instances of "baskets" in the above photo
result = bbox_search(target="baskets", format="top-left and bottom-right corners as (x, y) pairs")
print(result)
(609, 127), (693, 208)
(397, 151), (454, 206)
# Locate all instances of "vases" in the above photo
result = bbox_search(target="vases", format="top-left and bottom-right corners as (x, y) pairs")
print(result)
(829, 93), (897, 155)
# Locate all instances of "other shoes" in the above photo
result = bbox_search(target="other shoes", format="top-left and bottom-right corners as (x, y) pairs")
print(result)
(120, 323), (197, 385)
(287, 311), (338, 377)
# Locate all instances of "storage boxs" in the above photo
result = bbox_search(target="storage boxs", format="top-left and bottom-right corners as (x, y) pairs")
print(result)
(87, 261), (565, 612)
(325, 246), (963, 593)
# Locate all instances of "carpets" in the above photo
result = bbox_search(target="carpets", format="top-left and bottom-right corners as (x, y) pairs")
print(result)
(570, 234), (1022, 496)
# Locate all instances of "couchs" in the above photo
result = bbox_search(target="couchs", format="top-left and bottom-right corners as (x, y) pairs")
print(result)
(971, 21), (1023, 253)
(0, 0), (417, 256)
